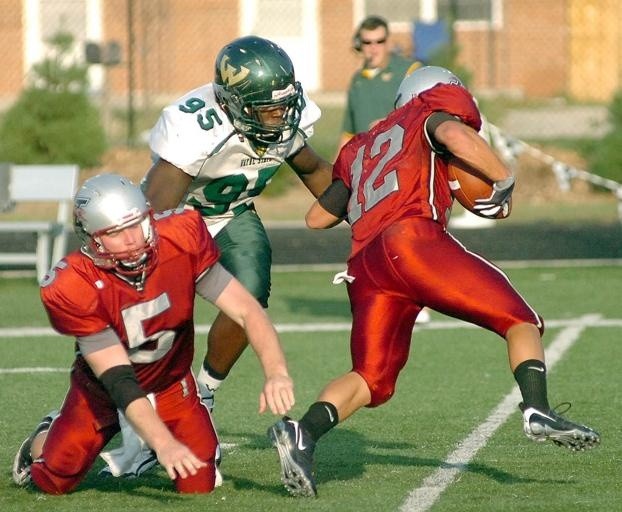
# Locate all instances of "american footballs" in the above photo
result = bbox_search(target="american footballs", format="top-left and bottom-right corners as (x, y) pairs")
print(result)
(448, 157), (511, 218)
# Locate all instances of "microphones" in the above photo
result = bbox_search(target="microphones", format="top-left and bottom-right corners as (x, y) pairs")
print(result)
(351, 46), (373, 63)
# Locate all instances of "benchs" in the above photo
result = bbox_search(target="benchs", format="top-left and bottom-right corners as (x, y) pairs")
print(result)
(0, 164), (79, 285)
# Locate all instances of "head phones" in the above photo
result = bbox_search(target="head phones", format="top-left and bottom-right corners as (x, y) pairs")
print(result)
(352, 15), (388, 52)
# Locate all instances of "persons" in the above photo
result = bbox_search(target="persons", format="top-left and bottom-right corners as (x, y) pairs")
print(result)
(333, 17), (464, 205)
(13, 173), (297, 498)
(264, 62), (602, 502)
(124, 36), (355, 454)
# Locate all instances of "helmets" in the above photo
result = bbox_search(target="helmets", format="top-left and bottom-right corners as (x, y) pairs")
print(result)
(213, 37), (306, 143)
(394, 66), (462, 110)
(74, 173), (159, 281)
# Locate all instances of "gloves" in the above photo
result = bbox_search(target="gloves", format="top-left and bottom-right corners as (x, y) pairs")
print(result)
(473, 179), (515, 218)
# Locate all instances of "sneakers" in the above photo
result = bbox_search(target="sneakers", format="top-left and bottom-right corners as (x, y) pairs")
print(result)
(13, 411), (59, 486)
(519, 401), (600, 452)
(267, 416), (317, 498)
(97, 449), (158, 480)
